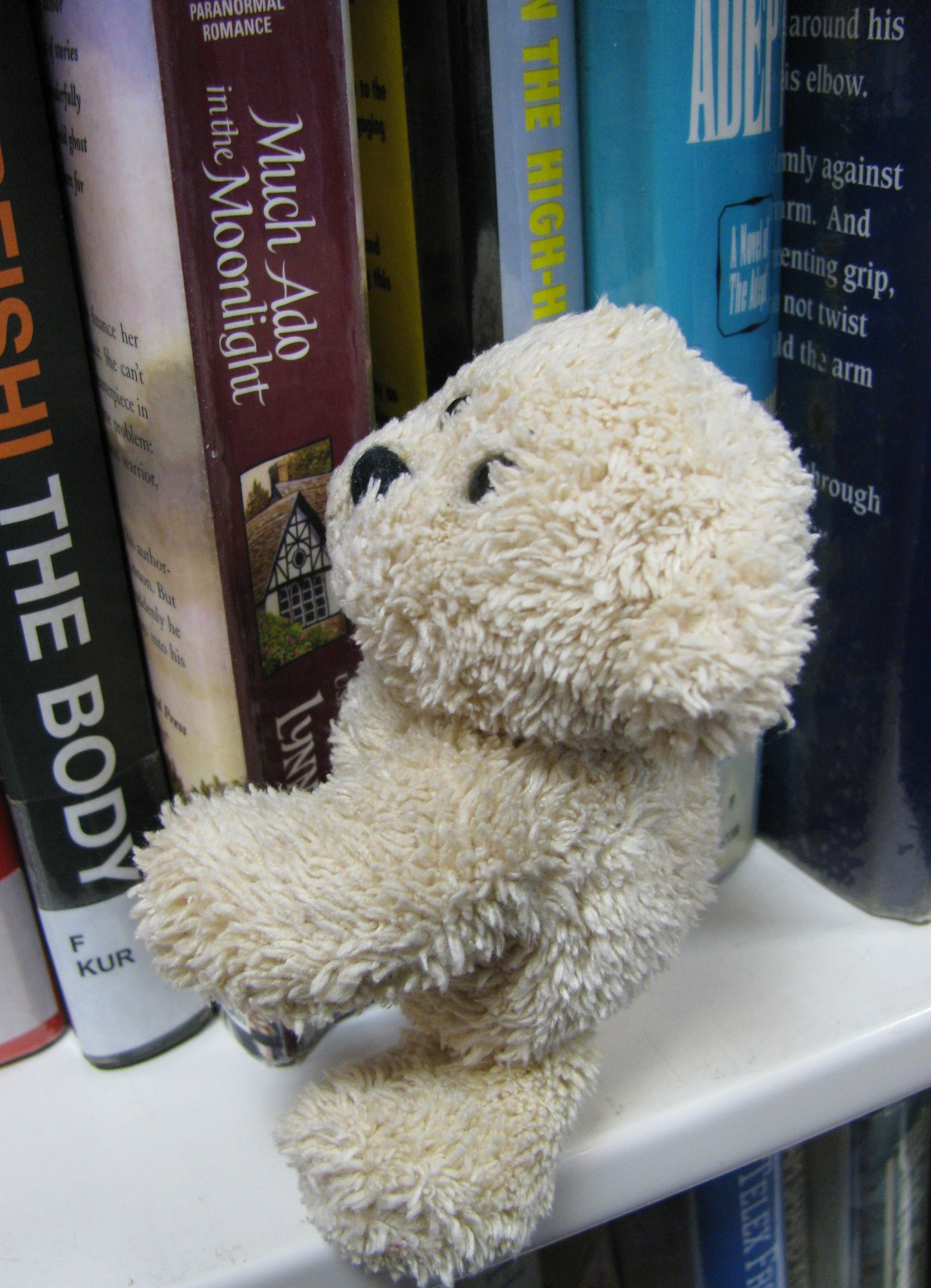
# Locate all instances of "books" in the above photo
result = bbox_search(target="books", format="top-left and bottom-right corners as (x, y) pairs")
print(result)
(0, 0), (931, 1288)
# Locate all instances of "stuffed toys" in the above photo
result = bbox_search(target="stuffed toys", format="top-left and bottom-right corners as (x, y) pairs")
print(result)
(124, 287), (826, 1288)
